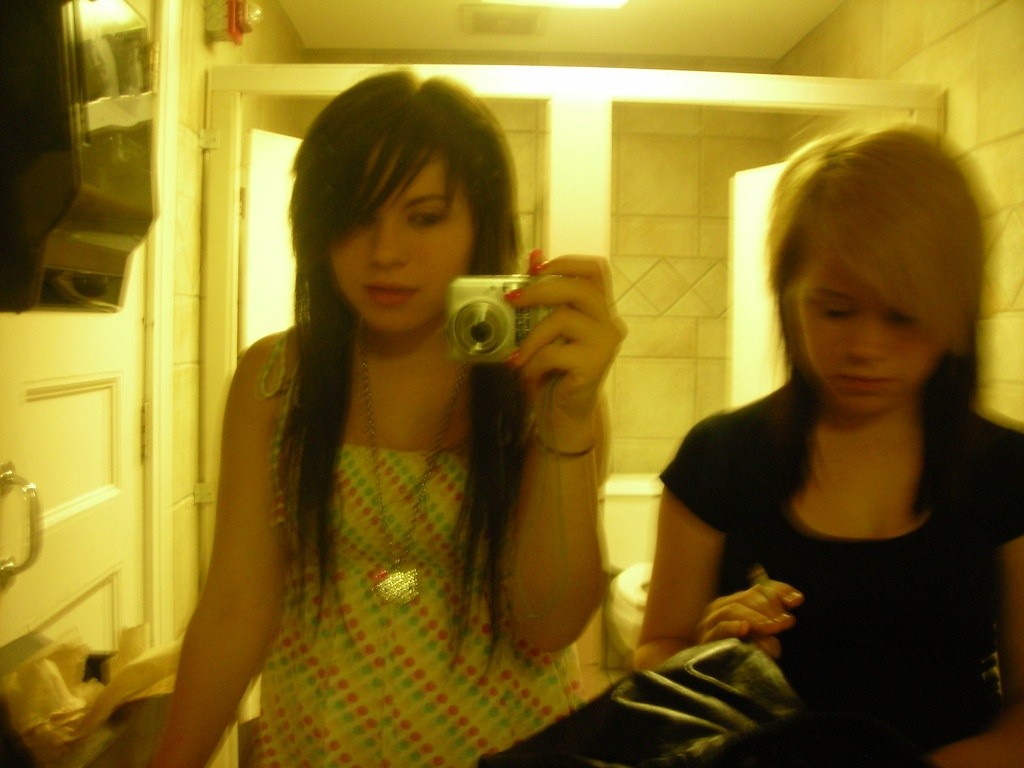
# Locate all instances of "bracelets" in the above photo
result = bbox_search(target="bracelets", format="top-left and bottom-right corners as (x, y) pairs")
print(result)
(524, 420), (598, 465)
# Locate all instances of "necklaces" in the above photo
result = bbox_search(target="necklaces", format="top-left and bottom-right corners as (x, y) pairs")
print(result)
(353, 323), (471, 608)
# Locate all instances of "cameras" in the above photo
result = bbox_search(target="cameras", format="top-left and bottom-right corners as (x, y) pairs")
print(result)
(444, 273), (568, 366)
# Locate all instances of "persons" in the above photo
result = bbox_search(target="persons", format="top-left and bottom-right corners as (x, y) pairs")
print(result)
(145, 64), (631, 768)
(634, 121), (1024, 768)
